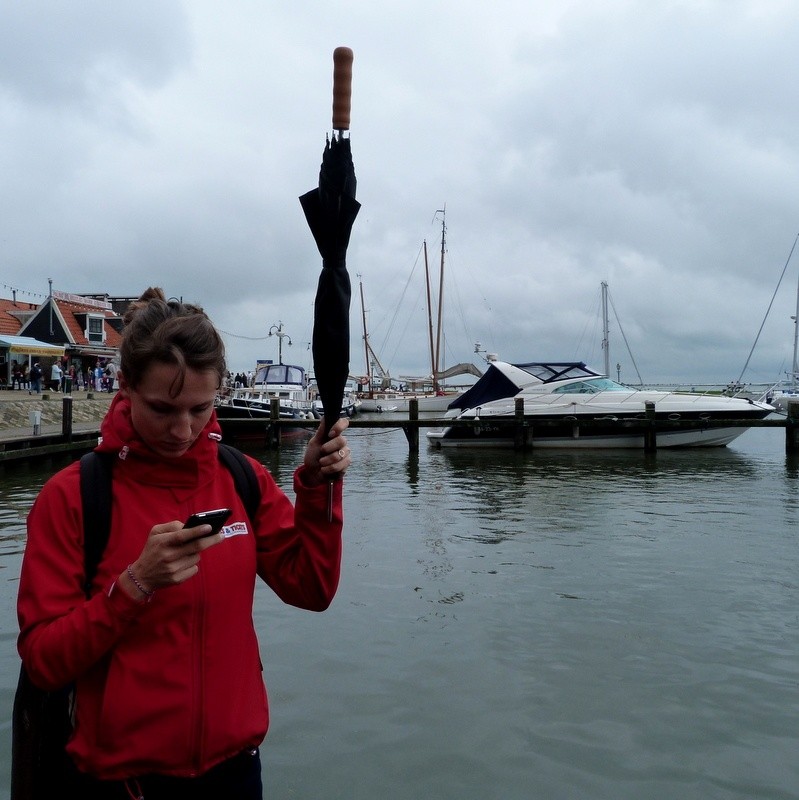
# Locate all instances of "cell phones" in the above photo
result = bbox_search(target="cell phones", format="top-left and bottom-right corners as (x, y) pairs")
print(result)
(180, 507), (233, 543)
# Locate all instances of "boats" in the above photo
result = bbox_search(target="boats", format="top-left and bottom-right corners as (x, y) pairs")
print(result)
(229, 362), (311, 418)
(421, 344), (779, 449)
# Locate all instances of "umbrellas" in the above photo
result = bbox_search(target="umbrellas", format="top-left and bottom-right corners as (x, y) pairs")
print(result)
(297, 47), (362, 442)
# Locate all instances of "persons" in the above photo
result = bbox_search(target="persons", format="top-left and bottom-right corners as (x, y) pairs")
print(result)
(11, 358), (119, 397)
(399, 382), (404, 392)
(226, 370), (253, 397)
(728, 381), (741, 391)
(13, 288), (352, 800)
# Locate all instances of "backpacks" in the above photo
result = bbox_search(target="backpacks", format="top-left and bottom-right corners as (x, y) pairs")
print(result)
(106, 365), (114, 376)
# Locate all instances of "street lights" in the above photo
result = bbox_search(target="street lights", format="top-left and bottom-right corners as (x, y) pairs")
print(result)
(267, 320), (292, 364)
(47, 277), (55, 336)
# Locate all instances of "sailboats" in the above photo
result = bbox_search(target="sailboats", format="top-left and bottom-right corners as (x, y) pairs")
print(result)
(307, 203), (473, 419)
(720, 229), (799, 409)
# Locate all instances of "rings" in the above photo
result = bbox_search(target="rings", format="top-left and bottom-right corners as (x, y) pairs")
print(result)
(338, 449), (346, 459)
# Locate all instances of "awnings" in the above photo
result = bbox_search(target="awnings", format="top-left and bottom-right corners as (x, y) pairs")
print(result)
(0, 334), (65, 357)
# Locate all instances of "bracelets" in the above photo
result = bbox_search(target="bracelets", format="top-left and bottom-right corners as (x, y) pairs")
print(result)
(127, 564), (152, 596)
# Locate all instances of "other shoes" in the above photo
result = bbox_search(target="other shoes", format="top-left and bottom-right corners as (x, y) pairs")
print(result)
(37, 392), (43, 396)
(29, 391), (32, 395)
(56, 391), (61, 393)
(49, 387), (55, 393)
